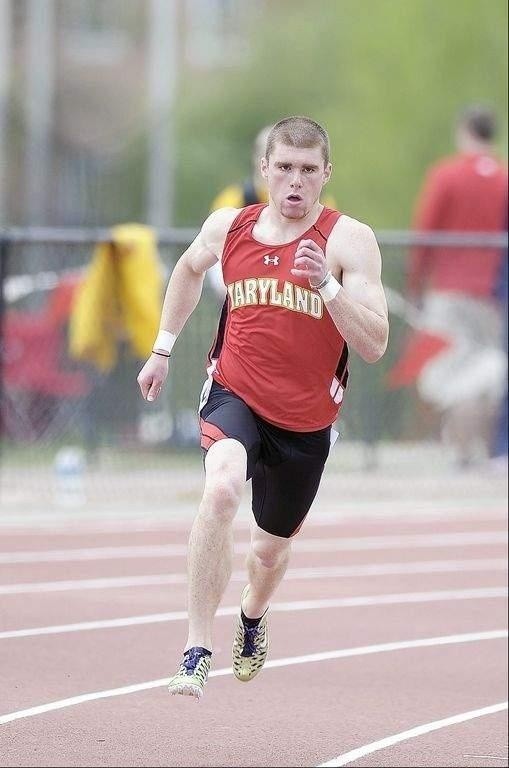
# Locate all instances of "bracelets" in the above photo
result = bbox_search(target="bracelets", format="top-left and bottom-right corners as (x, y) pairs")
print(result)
(152, 329), (177, 357)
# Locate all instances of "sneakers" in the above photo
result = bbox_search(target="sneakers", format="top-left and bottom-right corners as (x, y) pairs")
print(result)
(167, 646), (212, 700)
(232, 583), (270, 683)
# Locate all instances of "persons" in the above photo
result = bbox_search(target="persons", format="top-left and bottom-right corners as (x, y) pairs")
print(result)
(136, 116), (389, 699)
